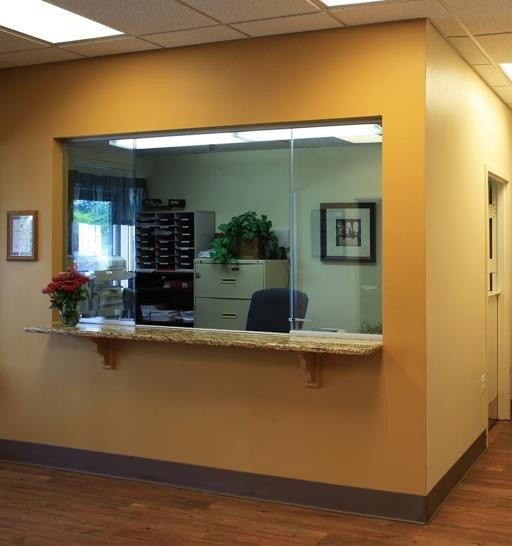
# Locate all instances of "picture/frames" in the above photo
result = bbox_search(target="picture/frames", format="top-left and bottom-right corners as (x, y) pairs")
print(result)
(5, 208), (37, 261)
(320, 201), (377, 262)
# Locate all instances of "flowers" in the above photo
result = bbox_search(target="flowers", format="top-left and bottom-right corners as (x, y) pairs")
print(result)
(40, 265), (93, 326)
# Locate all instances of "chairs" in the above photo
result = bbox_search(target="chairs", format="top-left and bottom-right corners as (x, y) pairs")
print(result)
(245, 288), (309, 334)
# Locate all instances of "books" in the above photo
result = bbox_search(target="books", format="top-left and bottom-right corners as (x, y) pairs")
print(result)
(141, 305), (176, 321)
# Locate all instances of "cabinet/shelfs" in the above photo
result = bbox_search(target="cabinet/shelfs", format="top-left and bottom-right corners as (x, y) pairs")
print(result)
(194, 258), (289, 331)
(134, 210), (215, 326)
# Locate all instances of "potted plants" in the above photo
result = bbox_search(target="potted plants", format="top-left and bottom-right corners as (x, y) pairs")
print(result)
(209, 208), (290, 265)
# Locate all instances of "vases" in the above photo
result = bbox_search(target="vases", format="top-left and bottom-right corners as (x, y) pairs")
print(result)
(58, 305), (82, 326)
